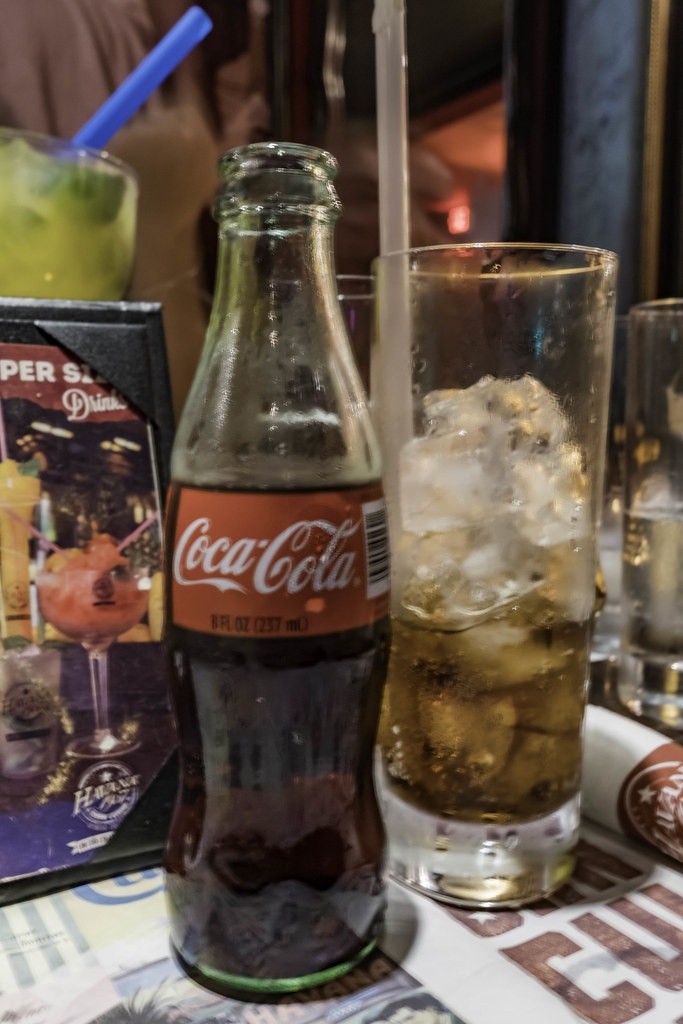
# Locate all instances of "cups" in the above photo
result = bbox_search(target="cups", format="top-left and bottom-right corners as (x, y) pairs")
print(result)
(0, 127), (139, 304)
(372, 239), (618, 914)
(622, 300), (683, 728)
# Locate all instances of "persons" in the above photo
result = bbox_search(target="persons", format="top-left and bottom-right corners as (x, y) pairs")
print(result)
(0, 0), (266, 430)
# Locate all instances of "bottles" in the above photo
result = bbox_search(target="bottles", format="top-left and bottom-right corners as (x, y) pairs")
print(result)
(166, 143), (386, 998)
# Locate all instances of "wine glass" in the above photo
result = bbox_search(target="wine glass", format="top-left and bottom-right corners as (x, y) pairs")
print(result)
(34, 571), (150, 760)
